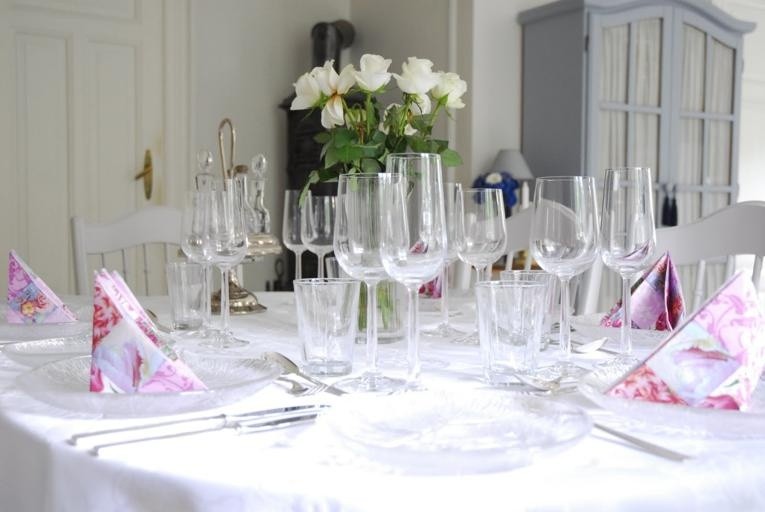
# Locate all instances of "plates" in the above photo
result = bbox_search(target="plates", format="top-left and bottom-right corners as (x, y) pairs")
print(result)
(576, 362), (765, 440)
(338, 398), (590, 473)
(1, 334), (285, 413)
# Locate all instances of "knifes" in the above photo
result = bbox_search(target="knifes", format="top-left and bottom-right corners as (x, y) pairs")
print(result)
(70, 402), (330, 456)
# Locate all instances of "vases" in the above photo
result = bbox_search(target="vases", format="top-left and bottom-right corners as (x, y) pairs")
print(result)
(347, 235), (423, 348)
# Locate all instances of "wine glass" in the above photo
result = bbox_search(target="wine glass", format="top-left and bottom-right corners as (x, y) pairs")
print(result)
(453, 186), (510, 283)
(420, 180), (466, 340)
(598, 166), (659, 363)
(333, 173), (411, 396)
(530, 172), (601, 377)
(379, 151), (451, 397)
(202, 180), (250, 345)
(281, 189), (314, 278)
(298, 194), (347, 278)
(177, 177), (223, 331)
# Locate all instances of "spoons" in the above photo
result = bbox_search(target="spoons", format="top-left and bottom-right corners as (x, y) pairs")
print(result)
(145, 309), (172, 333)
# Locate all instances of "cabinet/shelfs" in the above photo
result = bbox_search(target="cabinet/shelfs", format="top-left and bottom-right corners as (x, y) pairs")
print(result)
(517, 0), (757, 209)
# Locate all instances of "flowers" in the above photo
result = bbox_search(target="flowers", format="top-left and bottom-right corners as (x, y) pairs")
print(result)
(280, 52), (464, 330)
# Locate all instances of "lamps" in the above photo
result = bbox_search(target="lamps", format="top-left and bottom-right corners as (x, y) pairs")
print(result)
(491, 147), (534, 183)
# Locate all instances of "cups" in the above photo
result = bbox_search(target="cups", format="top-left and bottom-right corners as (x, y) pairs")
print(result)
(500, 269), (556, 352)
(475, 280), (547, 391)
(291, 277), (358, 377)
(167, 261), (212, 332)
(324, 255), (407, 344)
